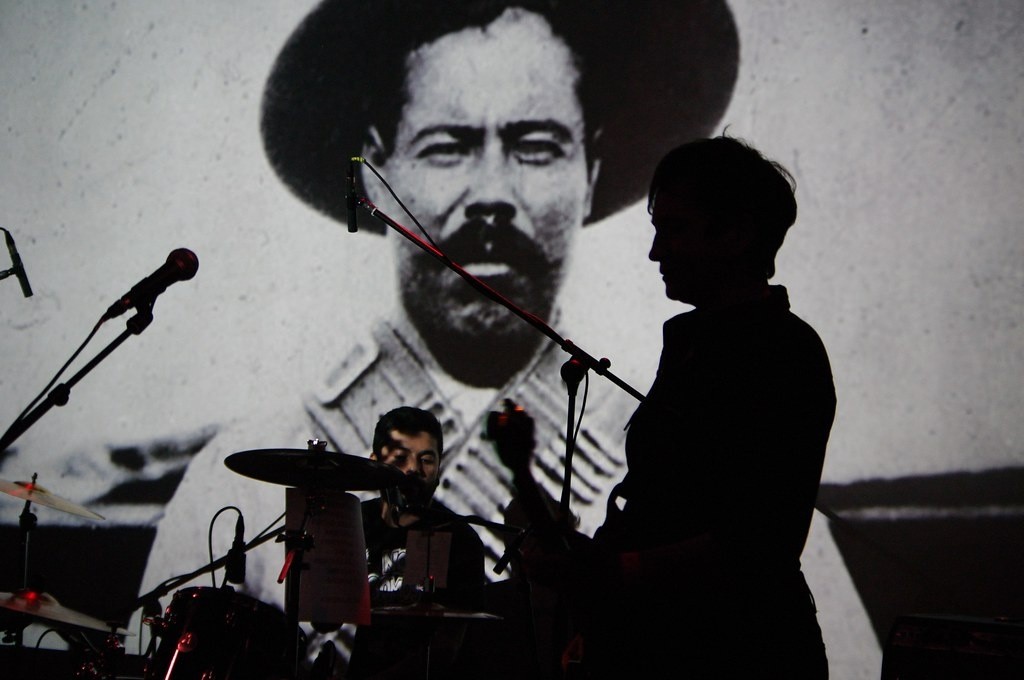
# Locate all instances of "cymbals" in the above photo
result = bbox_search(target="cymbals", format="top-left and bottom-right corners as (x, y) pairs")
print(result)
(223, 437), (406, 492)
(0, 471), (107, 521)
(0, 588), (139, 638)
(369, 589), (506, 622)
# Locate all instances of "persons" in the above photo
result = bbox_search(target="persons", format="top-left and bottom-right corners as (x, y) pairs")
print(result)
(360, 406), (484, 604)
(124, 0), (882, 680)
(522, 140), (835, 680)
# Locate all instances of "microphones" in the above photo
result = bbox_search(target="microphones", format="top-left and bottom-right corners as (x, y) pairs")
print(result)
(103, 248), (200, 320)
(345, 167), (359, 232)
(225, 515), (247, 584)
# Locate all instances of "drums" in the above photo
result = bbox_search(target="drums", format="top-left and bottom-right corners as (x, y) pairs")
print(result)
(147, 583), (310, 680)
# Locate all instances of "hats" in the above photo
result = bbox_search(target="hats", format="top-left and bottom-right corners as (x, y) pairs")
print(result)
(258, 0), (739, 236)
